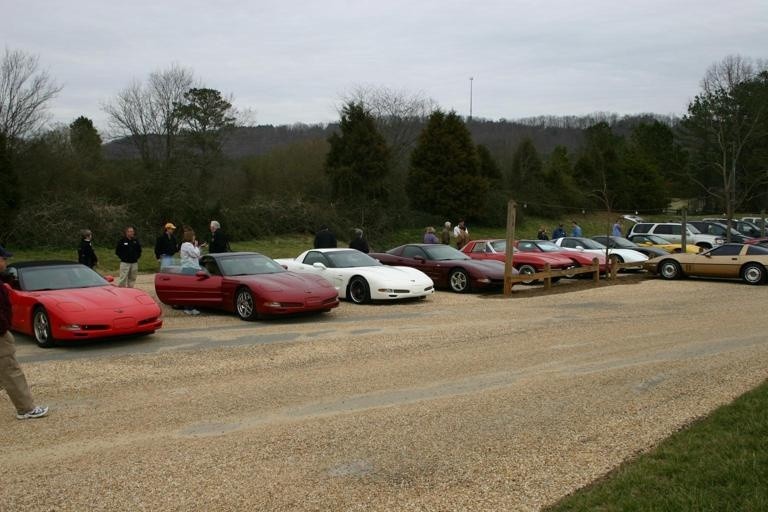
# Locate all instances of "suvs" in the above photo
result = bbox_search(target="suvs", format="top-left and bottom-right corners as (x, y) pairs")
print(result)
(616, 214), (768, 247)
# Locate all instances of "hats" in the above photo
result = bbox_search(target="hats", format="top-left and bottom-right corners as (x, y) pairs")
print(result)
(164, 222), (176, 229)
(320, 224), (328, 230)
(0, 245), (13, 258)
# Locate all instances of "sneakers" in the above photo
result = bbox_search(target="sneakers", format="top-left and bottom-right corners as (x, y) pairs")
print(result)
(183, 309), (202, 315)
(16, 405), (49, 420)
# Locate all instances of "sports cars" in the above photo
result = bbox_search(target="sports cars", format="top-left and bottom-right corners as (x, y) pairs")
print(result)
(458, 240), (574, 284)
(155, 249), (340, 320)
(517, 239), (611, 280)
(628, 234), (706, 258)
(273, 248), (436, 305)
(3, 258), (163, 347)
(367, 242), (520, 294)
(594, 235), (669, 261)
(547, 236), (648, 275)
(642, 243), (768, 285)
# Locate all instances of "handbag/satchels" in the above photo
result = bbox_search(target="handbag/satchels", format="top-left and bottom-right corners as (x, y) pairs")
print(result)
(224, 237), (232, 252)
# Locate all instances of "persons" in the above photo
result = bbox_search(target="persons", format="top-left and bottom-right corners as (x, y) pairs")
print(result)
(314, 224), (337, 248)
(571, 221), (583, 237)
(154, 222), (180, 266)
(424, 218), (473, 250)
(349, 228), (369, 254)
(180, 226), (201, 315)
(209, 220), (228, 253)
(77, 229), (99, 269)
(537, 225), (548, 240)
(116, 224), (142, 288)
(552, 223), (567, 239)
(612, 220), (622, 236)
(0, 247), (48, 420)
